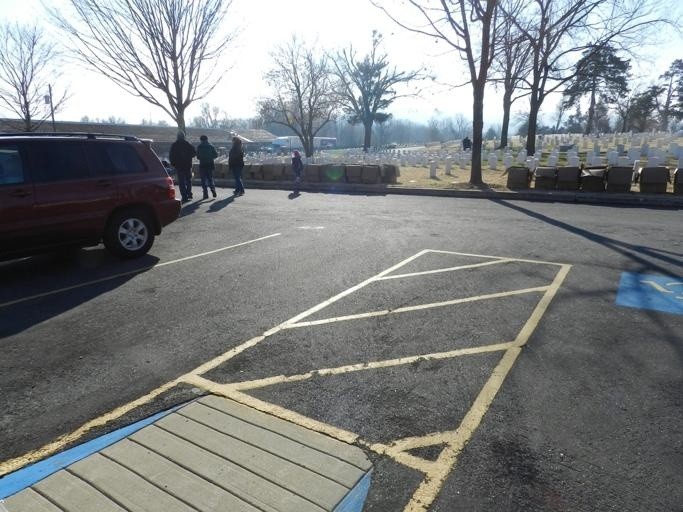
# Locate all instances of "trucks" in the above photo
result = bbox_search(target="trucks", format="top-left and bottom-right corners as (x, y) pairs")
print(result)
(272, 136), (338, 155)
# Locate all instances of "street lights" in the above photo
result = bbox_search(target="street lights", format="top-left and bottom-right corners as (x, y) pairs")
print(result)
(44, 84), (56, 133)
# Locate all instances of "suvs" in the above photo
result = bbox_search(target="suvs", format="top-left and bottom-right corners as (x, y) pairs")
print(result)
(0, 135), (179, 264)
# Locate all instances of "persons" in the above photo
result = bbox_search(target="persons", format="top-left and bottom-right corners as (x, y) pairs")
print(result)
(196, 135), (219, 198)
(291, 150), (304, 184)
(229, 137), (246, 197)
(169, 131), (196, 202)
